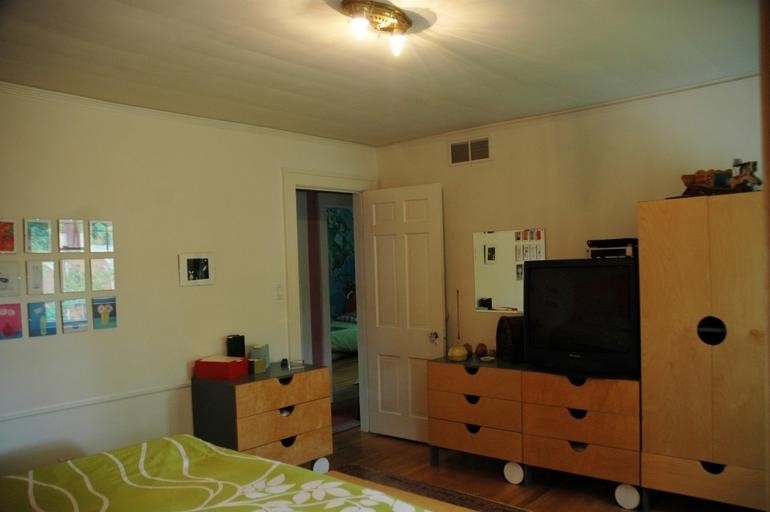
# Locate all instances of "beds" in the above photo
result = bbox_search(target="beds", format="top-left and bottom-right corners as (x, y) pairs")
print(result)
(1, 433), (437, 512)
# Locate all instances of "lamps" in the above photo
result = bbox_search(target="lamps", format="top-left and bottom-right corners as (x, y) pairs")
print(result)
(339, 0), (410, 57)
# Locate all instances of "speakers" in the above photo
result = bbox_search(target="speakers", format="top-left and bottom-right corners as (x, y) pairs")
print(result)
(495, 315), (524, 365)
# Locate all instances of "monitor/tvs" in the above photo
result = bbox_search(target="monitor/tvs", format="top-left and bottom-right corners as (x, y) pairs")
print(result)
(522, 257), (636, 375)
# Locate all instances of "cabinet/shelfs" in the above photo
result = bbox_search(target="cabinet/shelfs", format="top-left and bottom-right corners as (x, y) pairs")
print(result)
(635, 189), (769, 510)
(528, 369), (639, 503)
(426, 356), (523, 485)
(196, 364), (334, 466)
